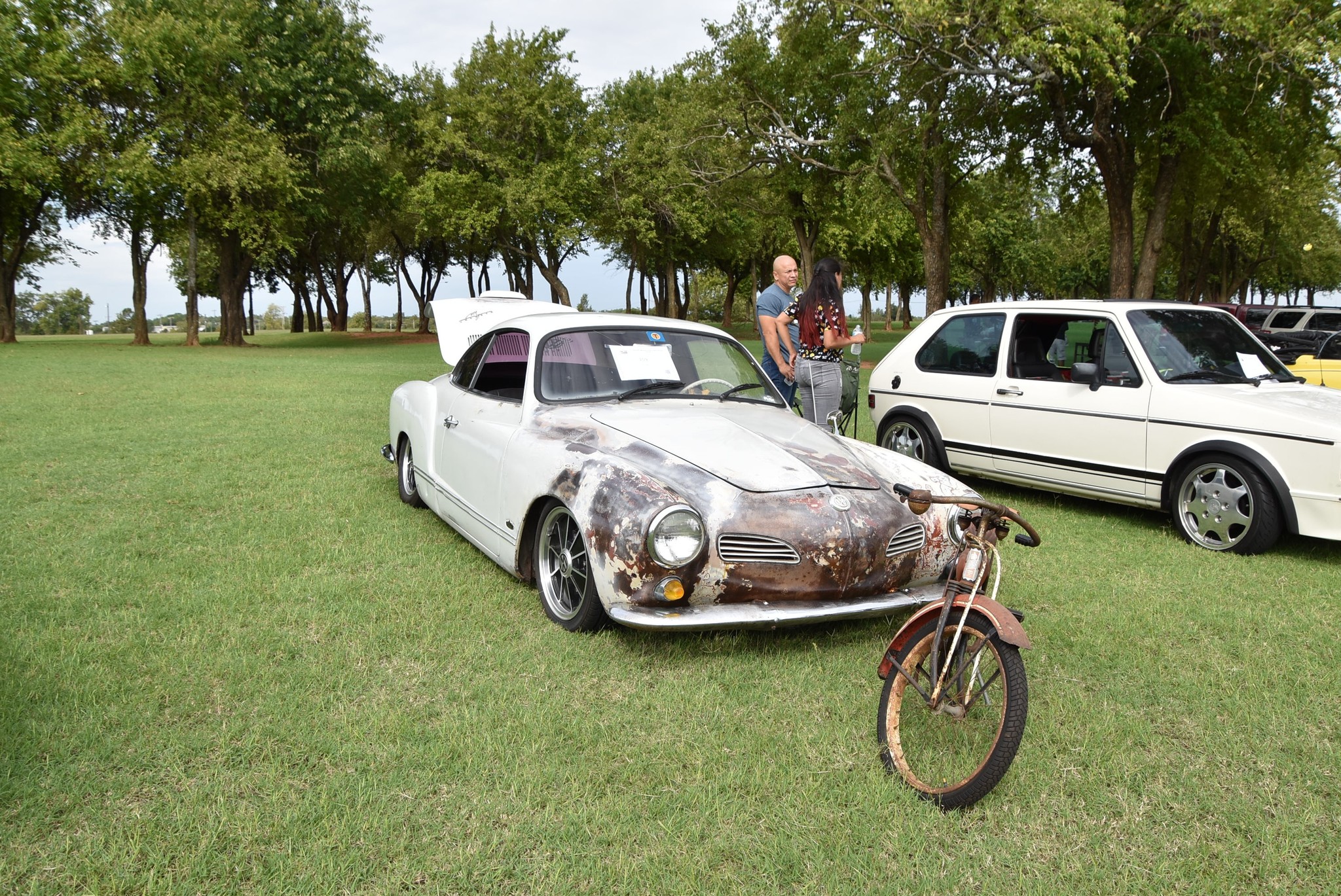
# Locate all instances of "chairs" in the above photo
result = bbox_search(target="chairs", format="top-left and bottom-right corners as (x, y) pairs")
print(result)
(1013, 336), (1066, 381)
(1085, 328), (1138, 387)
(792, 359), (860, 440)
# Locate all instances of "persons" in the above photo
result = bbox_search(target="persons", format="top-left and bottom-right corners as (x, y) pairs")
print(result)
(775, 257), (866, 433)
(755, 255), (800, 410)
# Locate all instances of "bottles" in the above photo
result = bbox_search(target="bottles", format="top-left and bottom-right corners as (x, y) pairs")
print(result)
(784, 375), (795, 386)
(851, 325), (863, 355)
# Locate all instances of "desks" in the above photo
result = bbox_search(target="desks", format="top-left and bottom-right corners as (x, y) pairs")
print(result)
(1073, 342), (1103, 363)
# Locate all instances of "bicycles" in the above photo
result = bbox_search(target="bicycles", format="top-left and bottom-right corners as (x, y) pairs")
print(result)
(876, 483), (1042, 814)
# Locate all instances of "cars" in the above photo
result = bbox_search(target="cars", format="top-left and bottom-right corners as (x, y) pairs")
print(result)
(382, 289), (1001, 635)
(869, 298), (1341, 560)
(1194, 303), (1341, 391)
